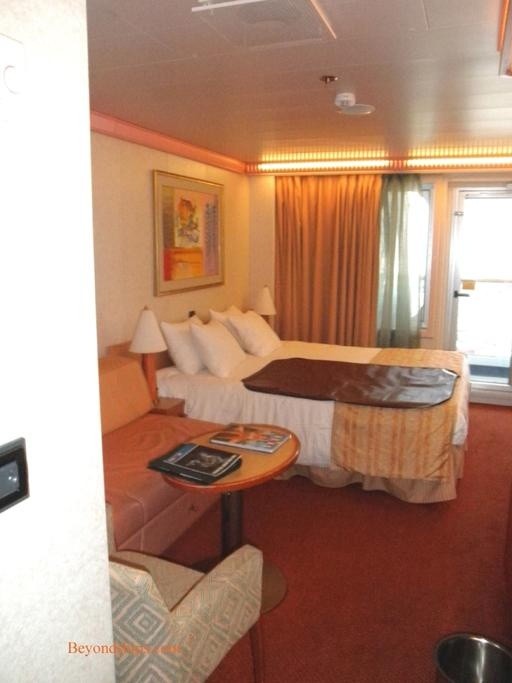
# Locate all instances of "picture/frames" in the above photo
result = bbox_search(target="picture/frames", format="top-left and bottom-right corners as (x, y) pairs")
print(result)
(154, 170), (225, 295)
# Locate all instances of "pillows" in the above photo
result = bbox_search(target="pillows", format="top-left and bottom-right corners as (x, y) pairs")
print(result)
(193, 319), (246, 378)
(209, 304), (245, 351)
(160, 314), (206, 373)
(227, 308), (281, 355)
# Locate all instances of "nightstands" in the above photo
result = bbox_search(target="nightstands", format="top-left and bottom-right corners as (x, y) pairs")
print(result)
(146, 395), (187, 416)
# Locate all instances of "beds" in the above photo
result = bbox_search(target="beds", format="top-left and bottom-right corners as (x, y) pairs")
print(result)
(106, 318), (469, 505)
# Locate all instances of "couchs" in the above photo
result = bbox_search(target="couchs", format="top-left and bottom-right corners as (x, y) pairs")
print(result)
(98, 356), (233, 557)
(103, 502), (264, 682)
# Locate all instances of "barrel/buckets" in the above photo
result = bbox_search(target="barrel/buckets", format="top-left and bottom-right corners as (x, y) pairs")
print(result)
(434, 632), (511, 683)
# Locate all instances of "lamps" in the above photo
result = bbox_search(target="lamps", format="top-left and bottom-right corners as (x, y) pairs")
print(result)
(129, 306), (168, 408)
(252, 286), (276, 333)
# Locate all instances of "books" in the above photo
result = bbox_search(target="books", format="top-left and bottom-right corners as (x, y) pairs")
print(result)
(210, 422), (292, 453)
(161, 444), (243, 477)
